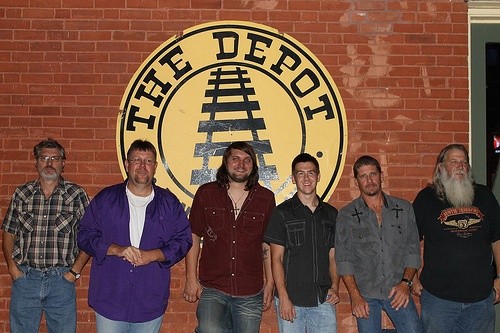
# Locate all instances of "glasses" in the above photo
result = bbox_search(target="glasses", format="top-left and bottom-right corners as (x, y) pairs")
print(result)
(37, 155), (63, 161)
(128, 157), (156, 164)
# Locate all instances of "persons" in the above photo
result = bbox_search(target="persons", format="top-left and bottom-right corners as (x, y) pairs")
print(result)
(335, 156), (425, 333)
(183, 142), (276, 333)
(264, 153), (340, 333)
(2, 139), (89, 333)
(412, 144), (500, 333)
(78, 141), (193, 333)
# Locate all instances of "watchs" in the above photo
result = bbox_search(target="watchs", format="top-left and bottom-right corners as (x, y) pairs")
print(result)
(402, 278), (412, 288)
(70, 269), (80, 279)
